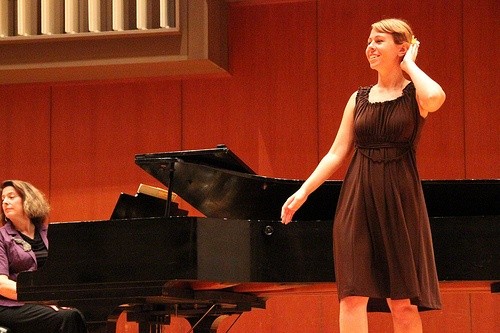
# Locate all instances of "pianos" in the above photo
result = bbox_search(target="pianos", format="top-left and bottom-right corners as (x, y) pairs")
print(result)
(16, 144), (500, 325)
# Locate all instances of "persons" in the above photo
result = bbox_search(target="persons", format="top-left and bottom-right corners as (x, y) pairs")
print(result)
(279, 18), (447, 333)
(0, 180), (90, 333)
